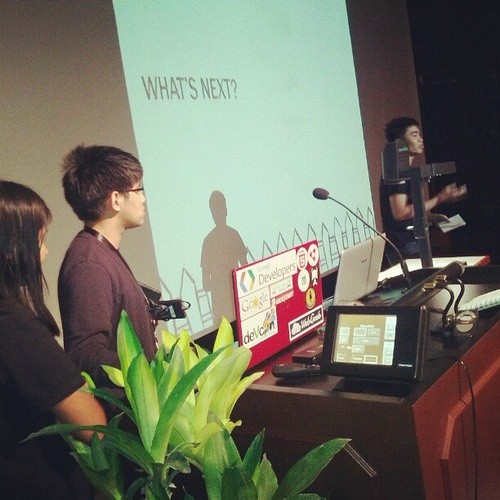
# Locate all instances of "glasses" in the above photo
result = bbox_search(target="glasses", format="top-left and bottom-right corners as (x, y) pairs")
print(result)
(105, 185), (144, 202)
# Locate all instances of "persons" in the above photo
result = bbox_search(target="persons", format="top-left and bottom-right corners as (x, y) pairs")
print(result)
(57, 142), (160, 434)
(379, 116), (469, 267)
(0, 180), (106, 500)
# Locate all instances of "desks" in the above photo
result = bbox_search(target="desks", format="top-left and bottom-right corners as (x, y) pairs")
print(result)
(235, 282), (500, 500)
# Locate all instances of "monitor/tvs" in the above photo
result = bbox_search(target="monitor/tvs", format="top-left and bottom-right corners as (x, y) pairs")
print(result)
(320, 307), (429, 392)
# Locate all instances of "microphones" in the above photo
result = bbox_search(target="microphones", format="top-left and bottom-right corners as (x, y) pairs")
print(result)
(313, 188), (412, 290)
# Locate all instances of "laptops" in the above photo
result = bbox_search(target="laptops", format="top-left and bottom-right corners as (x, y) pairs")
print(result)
(332, 233), (387, 306)
